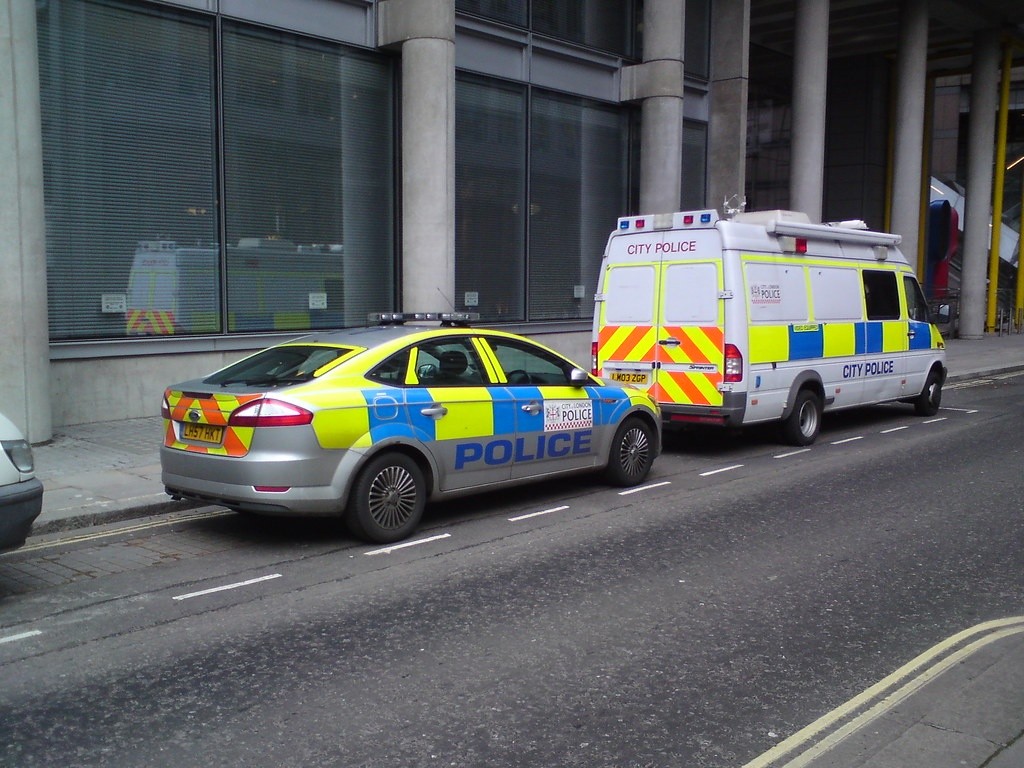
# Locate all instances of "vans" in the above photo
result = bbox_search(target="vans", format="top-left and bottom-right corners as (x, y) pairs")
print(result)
(592, 195), (952, 448)
(124, 236), (345, 338)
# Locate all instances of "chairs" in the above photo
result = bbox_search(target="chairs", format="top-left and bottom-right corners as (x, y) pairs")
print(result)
(374, 352), (402, 383)
(438, 350), (480, 384)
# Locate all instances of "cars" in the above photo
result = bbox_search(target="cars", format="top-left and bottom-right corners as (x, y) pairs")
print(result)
(159, 309), (663, 546)
